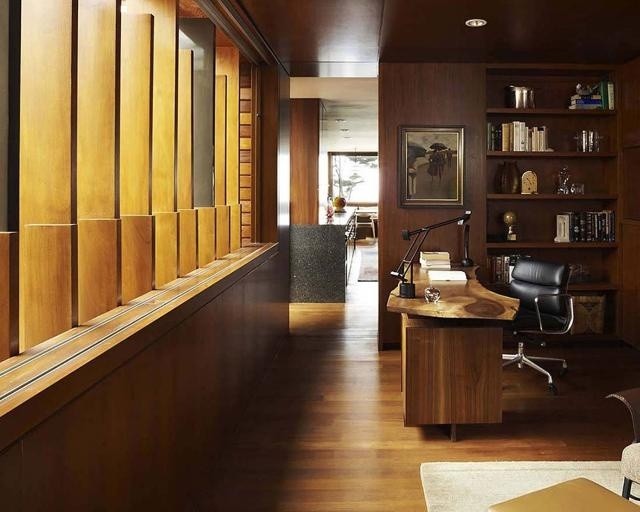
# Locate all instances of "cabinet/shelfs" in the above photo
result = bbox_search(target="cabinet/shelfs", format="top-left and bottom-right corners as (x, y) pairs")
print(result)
(484, 67), (621, 342)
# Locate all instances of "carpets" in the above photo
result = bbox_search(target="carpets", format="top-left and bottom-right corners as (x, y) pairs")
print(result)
(358, 251), (378, 282)
(420, 461), (640, 512)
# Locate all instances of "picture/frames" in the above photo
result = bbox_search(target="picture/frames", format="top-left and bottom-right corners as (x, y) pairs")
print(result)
(398, 126), (466, 208)
(328, 152), (379, 206)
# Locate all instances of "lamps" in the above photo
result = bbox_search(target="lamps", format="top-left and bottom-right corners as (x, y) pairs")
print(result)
(391, 209), (472, 299)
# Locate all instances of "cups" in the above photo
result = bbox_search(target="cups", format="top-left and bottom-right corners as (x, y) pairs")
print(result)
(589, 129), (600, 153)
(570, 183), (584, 196)
(577, 130), (588, 153)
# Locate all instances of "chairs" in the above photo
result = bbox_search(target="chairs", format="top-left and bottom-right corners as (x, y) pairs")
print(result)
(501, 258), (575, 394)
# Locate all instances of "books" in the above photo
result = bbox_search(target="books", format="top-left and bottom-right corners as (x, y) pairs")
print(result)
(556, 207), (615, 243)
(483, 86), (608, 154)
(419, 250), (450, 270)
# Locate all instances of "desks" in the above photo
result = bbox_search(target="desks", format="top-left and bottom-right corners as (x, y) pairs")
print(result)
(386, 263), (520, 443)
